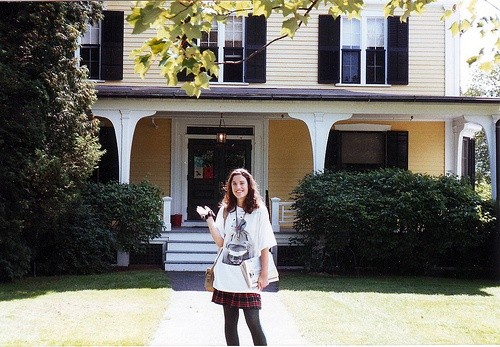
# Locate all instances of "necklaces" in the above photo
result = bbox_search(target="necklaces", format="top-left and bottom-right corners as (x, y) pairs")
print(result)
(235, 204), (247, 240)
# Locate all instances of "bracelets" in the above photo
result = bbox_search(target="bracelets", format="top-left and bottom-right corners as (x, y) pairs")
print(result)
(200, 210), (216, 221)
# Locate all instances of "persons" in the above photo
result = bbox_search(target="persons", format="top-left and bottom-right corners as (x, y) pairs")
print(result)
(197, 168), (279, 346)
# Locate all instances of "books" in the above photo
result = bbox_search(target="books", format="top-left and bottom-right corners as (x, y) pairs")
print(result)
(240, 252), (279, 289)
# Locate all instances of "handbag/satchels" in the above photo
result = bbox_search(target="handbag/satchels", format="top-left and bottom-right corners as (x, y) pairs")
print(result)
(204, 268), (215, 291)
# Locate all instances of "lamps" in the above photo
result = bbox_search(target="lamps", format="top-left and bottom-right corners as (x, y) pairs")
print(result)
(216, 114), (226, 143)
